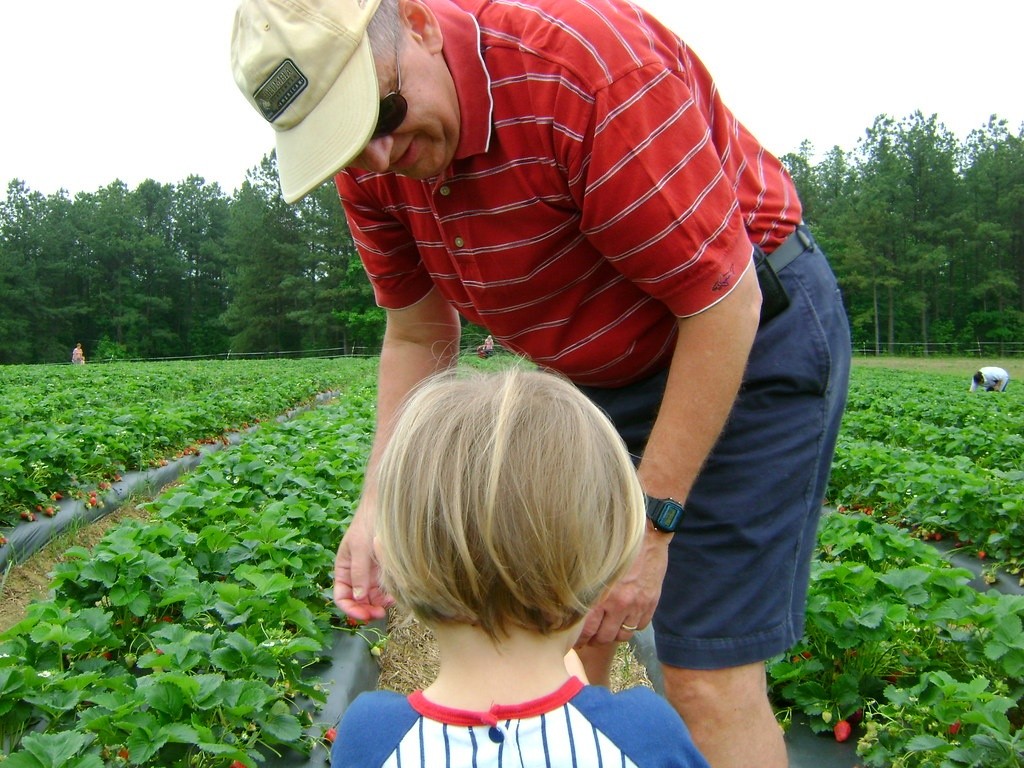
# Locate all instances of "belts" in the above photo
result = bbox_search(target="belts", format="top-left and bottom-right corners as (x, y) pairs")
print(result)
(768, 226), (811, 270)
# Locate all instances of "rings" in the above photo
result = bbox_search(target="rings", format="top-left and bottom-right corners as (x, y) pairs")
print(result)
(621, 624), (638, 632)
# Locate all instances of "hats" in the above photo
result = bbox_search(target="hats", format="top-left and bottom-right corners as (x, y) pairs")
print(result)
(231, 0), (381, 205)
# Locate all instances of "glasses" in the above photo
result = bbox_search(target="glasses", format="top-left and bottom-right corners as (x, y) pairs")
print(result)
(372, 48), (408, 141)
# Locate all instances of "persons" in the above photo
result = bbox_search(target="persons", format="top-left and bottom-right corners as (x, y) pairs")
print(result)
(232, 1), (853, 768)
(71, 342), (85, 365)
(333, 363), (708, 768)
(476, 334), (494, 359)
(968, 366), (1009, 393)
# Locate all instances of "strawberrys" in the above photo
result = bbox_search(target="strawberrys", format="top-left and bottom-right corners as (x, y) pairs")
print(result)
(98, 613), (175, 760)
(839, 504), (1024, 588)
(344, 615), (387, 656)
(792, 651), (962, 741)
(0, 416), (257, 547)
(326, 728), (337, 742)
(229, 759), (248, 768)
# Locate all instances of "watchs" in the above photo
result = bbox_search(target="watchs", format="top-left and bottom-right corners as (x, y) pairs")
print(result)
(643, 495), (686, 533)
